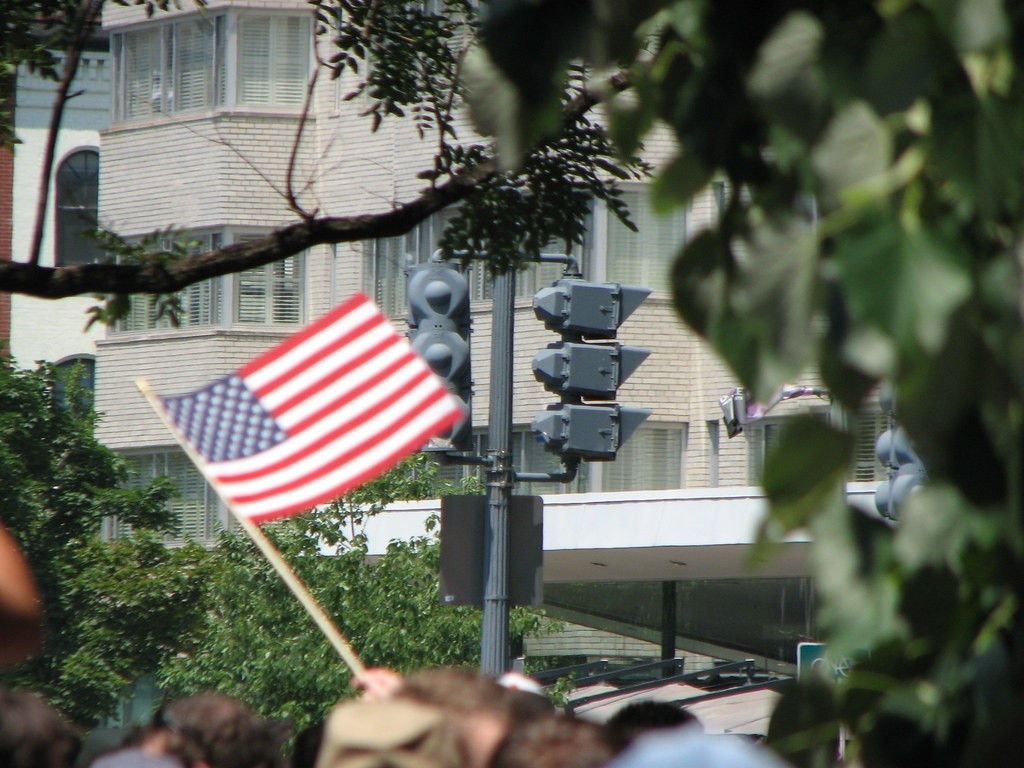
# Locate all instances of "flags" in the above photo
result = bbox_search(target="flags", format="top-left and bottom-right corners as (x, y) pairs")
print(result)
(154, 288), (468, 525)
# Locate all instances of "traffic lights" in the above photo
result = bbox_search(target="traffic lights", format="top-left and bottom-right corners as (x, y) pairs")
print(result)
(530, 278), (656, 463)
(873, 381), (931, 522)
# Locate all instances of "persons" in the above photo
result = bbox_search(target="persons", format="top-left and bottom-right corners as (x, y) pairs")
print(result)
(0, 667), (797, 768)
